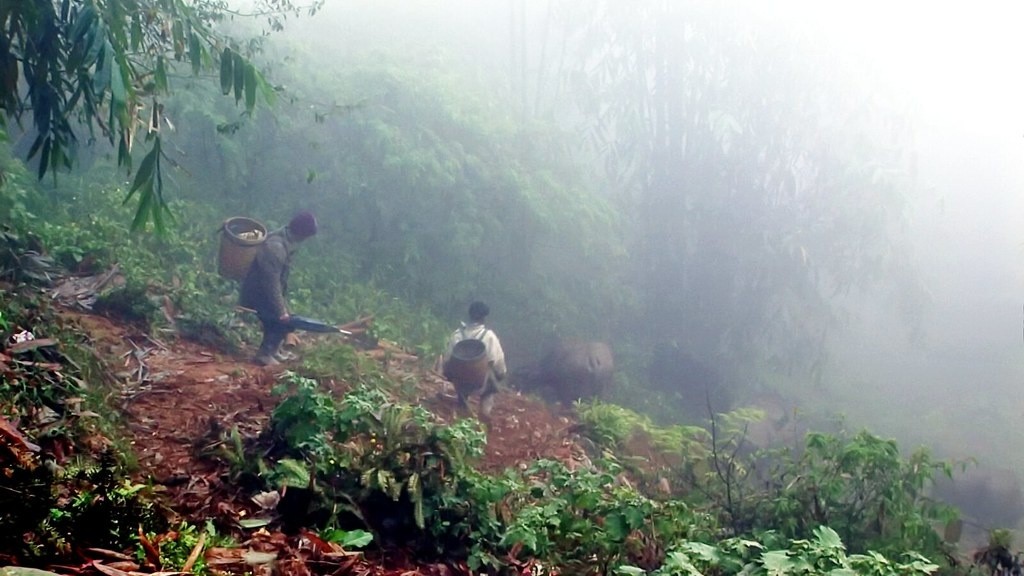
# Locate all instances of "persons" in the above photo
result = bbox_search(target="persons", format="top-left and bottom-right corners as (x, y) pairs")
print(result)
(444, 301), (507, 422)
(242, 214), (318, 365)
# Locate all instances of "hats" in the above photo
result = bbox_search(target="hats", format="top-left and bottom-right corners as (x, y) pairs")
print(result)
(290, 214), (318, 236)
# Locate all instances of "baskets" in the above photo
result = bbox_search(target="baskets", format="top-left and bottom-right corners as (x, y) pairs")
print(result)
(218, 216), (266, 281)
(449, 339), (489, 392)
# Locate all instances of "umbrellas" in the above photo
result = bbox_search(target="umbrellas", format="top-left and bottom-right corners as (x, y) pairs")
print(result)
(235, 305), (351, 341)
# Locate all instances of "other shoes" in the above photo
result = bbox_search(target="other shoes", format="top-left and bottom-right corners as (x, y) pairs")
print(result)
(273, 351), (289, 363)
(253, 352), (280, 364)
